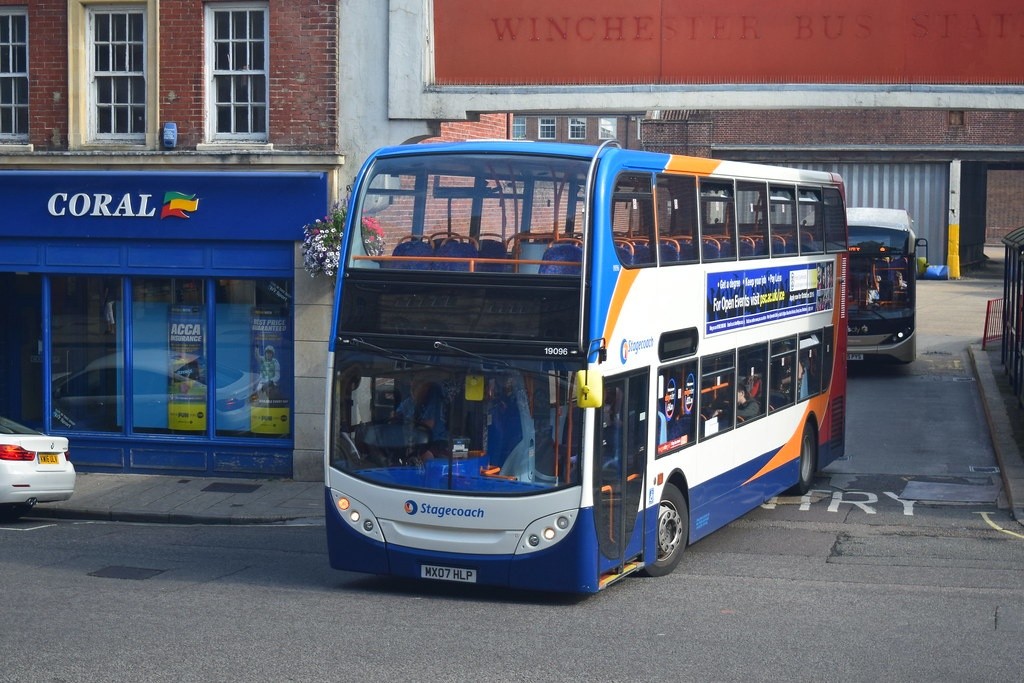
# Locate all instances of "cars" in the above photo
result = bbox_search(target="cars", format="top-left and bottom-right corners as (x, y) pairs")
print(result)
(0, 415), (77, 522)
(53, 348), (270, 436)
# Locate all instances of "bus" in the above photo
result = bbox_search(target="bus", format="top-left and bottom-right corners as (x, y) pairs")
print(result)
(323, 140), (849, 594)
(799, 206), (929, 366)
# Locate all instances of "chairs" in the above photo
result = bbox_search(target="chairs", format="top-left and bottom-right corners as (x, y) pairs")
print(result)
(669, 413), (706, 441)
(390, 227), (814, 276)
(657, 411), (667, 445)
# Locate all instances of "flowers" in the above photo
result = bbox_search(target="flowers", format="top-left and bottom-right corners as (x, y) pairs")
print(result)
(298, 193), (386, 288)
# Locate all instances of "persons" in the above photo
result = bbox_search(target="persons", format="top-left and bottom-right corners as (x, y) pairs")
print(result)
(99, 276), (117, 336)
(875, 248), (909, 265)
(867, 275), (890, 304)
(889, 271), (908, 291)
(710, 383), (762, 425)
(773, 344), (805, 404)
(389, 375), (438, 463)
(254, 339), (281, 402)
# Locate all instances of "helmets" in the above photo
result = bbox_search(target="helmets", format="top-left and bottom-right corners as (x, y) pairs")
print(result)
(264, 345), (275, 354)
